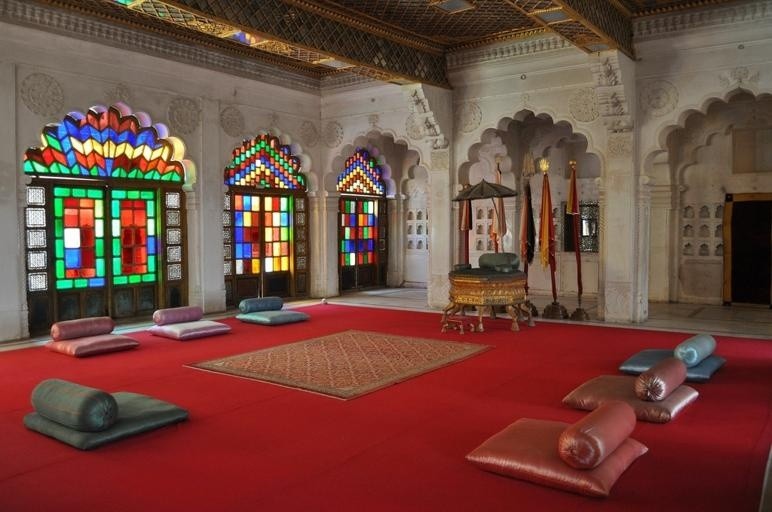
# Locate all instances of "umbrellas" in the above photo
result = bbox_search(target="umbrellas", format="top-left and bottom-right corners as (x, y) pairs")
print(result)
(451, 178), (518, 256)
(538, 171), (563, 302)
(458, 182), (473, 264)
(518, 178), (537, 297)
(566, 160), (589, 308)
(490, 162), (509, 252)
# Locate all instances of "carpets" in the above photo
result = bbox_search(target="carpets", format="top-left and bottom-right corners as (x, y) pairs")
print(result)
(182, 328), (497, 403)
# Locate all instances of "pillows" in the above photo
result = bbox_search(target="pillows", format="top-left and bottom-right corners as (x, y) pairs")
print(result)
(22, 374), (188, 451)
(43, 296), (313, 358)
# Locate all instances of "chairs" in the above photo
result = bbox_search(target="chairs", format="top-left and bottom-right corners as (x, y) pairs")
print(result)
(454, 252), (520, 321)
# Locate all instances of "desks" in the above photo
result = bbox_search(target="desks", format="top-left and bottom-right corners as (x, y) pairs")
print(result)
(440, 267), (536, 333)
